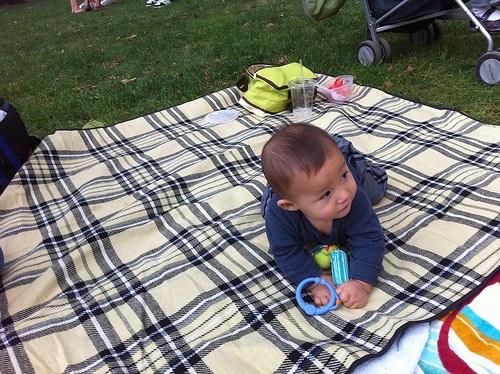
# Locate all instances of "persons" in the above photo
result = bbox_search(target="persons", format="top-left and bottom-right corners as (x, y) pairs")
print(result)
(70, 0), (85, 13)
(84, 0), (104, 11)
(261, 123), (388, 309)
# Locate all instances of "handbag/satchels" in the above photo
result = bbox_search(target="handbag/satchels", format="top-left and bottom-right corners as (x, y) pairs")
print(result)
(235, 62), (318, 117)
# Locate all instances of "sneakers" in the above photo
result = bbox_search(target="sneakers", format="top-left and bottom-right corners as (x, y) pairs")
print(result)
(146, 0), (174, 8)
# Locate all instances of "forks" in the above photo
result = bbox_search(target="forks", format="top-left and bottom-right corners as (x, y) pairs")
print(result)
(308, 80), (348, 102)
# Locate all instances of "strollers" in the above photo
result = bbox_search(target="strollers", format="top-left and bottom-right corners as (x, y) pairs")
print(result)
(355, 0), (500, 87)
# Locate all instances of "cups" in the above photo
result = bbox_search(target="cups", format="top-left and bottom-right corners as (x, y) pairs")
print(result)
(288, 78), (317, 121)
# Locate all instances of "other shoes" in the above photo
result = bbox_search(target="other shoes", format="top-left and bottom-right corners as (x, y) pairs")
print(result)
(79, 0), (116, 11)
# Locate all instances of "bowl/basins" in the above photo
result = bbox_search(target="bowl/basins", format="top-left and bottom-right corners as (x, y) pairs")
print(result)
(319, 75), (354, 104)
